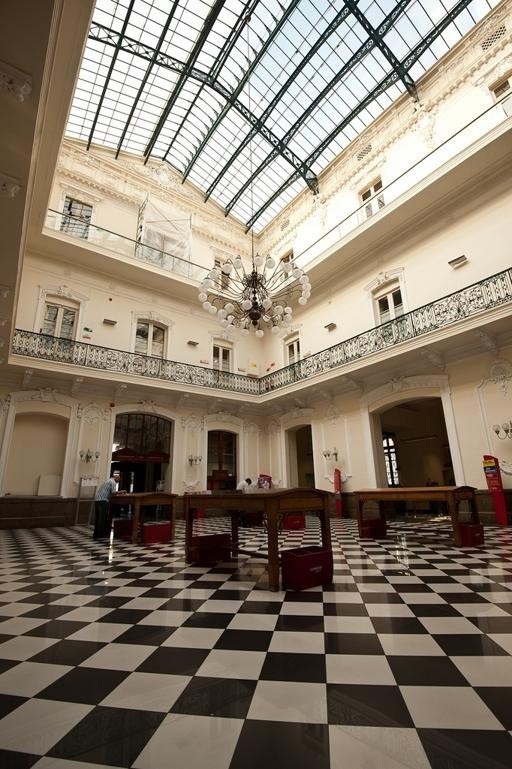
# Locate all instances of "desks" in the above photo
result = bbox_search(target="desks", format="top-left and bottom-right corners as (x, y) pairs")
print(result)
(352, 486), (479, 546)
(110, 486), (334, 592)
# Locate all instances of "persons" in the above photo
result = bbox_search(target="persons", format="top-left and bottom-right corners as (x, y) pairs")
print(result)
(236, 479), (252, 490)
(93, 476), (127, 538)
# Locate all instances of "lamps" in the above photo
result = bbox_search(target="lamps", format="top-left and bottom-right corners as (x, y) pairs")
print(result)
(79, 447), (100, 464)
(321, 446), (338, 462)
(491, 420), (512, 440)
(187, 454), (203, 467)
(196, 15), (312, 341)
